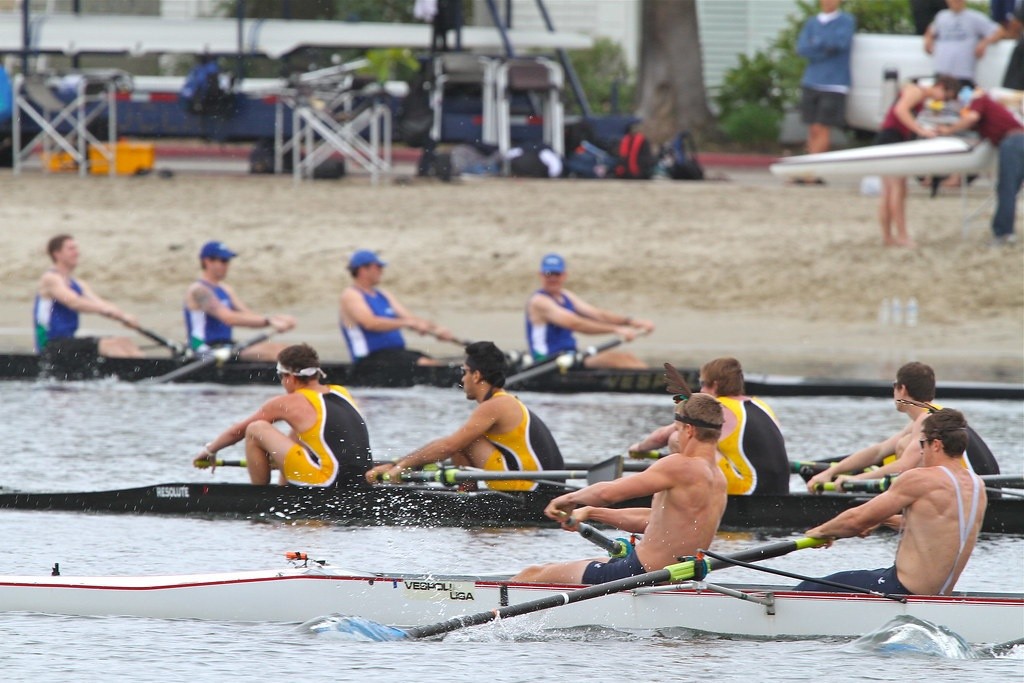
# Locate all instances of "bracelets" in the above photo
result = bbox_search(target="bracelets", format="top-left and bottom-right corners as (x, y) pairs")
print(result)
(383, 461), (396, 466)
(623, 315), (633, 324)
(204, 443), (215, 460)
(264, 314), (271, 326)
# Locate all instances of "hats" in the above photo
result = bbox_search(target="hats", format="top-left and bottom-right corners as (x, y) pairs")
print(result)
(539, 254), (564, 273)
(350, 249), (383, 270)
(201, 242), (235, 261)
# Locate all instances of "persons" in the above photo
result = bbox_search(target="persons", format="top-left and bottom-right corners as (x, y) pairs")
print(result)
(338, 250), (452, 364)
(524, 255), (654, 369)
(807, 362), (1002, 497)
(183, 241), (295, 362)
(504, 362), (729, 584)
(936, 78), (1024, 243)
(193, 341), (374, 489)
(794, 0), (855, 184)
(1000, 0), (1024, 91)
(32, 233), (146, 358)
(793, 407), (987, 596)
(873, 76), (959, 247)
(923, 0), (1005, 101)
(628, 355), (789, 495)
(365, 341), (566, 491)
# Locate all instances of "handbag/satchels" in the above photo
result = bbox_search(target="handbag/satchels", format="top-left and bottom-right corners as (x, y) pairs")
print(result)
(510, 121), (704, 179)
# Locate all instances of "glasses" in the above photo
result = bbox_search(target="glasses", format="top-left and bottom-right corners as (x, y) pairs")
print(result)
(920, 435), (940, 448)
(893, 381), (900, 389)
(460, 367), (469, 375)
(699, 380), (711, 387)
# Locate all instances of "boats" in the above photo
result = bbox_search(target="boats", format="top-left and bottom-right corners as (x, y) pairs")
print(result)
(769, 89), (1024, 180)
(0, 552), (1023, 649)
(0, 350), (1023, 402)
(0, 482), (1024, 537)
(0, 13), (641, 175)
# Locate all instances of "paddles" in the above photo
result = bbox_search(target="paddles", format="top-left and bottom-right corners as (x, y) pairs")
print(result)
(155, 326), (292, 383)
(633, 448), (874, 475)
(311, 530), (872, 642)
(555, 511), (637, 559)
(812, 472), (1023, 490)
(194, 456), (647, 473)
(427, 330), (518, 359)
(374, 464), (589, 479)
(117, 318), (183, 354)
(503, 328), (648, 388)
